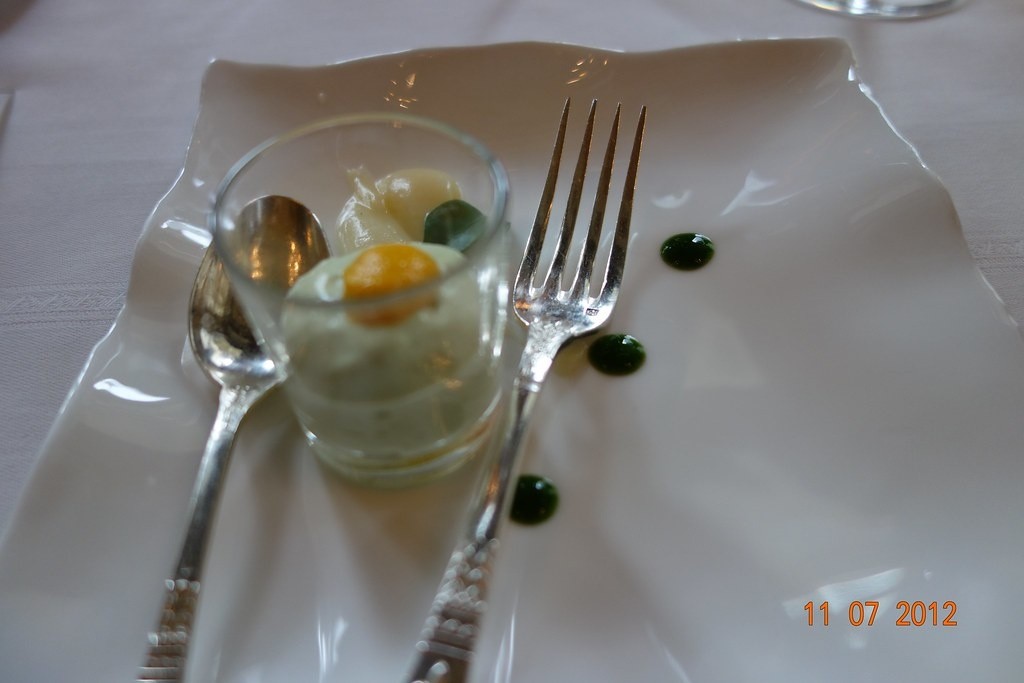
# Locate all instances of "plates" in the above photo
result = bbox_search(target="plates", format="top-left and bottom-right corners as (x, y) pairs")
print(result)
(0, 36), (1024, 683)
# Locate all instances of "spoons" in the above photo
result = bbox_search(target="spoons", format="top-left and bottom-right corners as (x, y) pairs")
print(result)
(136, 194), (331, 683)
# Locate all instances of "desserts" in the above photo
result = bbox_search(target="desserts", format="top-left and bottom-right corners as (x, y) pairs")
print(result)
(277, 237), (495, 485)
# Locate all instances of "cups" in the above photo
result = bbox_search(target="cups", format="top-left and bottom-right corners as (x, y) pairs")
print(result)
(213, 112), (513, 491)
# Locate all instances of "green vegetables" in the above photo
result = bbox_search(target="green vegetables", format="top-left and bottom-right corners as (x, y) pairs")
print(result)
(419, 198), (491, 252)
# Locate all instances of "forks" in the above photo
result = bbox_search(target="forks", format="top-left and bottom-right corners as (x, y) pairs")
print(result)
(407, 98), (649, 683)
